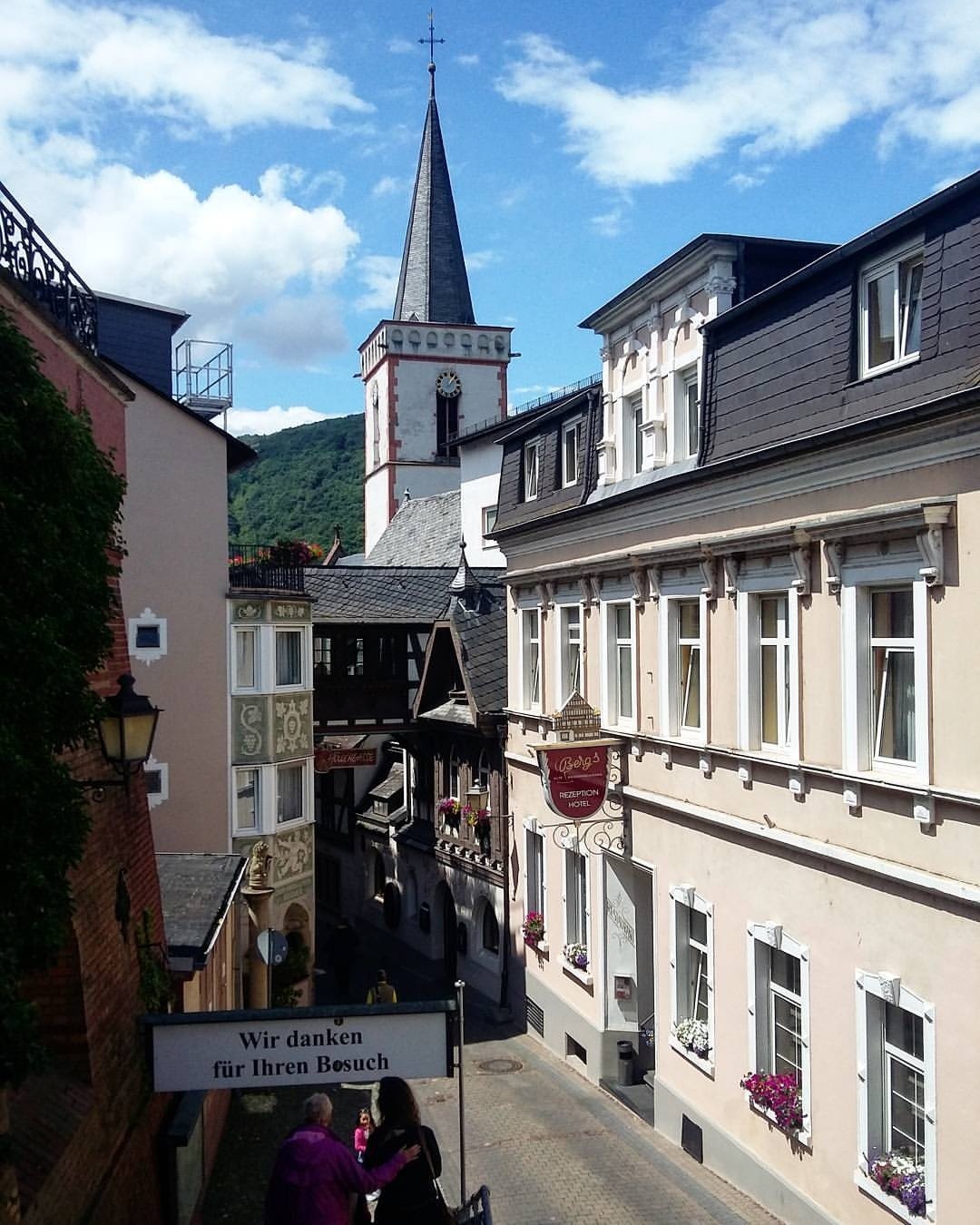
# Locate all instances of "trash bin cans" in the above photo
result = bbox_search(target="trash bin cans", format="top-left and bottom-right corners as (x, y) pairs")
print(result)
(616, 1041), (633, 1086)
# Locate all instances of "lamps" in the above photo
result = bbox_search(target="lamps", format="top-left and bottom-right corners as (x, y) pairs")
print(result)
(58, 673), (160, 811)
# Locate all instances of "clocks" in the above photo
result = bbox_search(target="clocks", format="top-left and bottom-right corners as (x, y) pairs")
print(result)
(372, 384), (379, 406)
(434, 369), (463, 397)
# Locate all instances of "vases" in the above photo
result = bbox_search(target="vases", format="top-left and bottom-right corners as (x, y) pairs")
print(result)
(445, 810), (458, 827)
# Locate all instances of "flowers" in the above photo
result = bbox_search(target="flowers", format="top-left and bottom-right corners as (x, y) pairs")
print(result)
(462, 806), (486, 826)
(675, 1017), (707, 1057)
(522, 913), (544, 944)
(563, 943), (587, 970)
(741, 1072), (802, 1128)
(229, 541), (323, 575)
(436, 797), (460, 814)
(872, 1154), (927, 1215)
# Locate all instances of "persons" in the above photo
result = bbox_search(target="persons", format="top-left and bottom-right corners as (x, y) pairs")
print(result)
(354, 1107), (377, 1164)
(360, 1076), (449, 1224)
(365, 969), (397, 1005)
(262, 1093), (421, 1224)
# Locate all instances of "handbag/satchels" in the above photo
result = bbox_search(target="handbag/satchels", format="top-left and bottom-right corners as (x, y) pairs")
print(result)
(437, 1189), (451, 1225)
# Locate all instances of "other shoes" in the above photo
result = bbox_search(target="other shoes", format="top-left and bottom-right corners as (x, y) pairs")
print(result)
(367, 1192), (380, 1201)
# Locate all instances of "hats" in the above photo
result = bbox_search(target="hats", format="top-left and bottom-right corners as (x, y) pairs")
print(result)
(377, 969), (386, 980)
